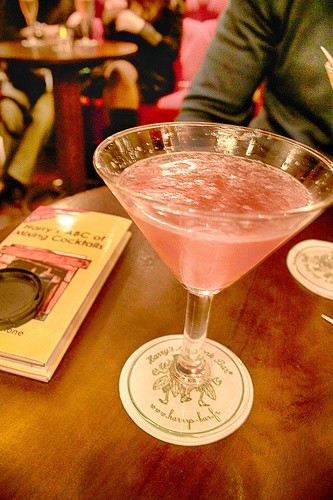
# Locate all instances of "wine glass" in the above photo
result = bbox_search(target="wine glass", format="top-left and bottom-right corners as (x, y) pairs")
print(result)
(18, 0), (41, 47)
(92, 122), (333, 447)
(74, 0), (97, 47)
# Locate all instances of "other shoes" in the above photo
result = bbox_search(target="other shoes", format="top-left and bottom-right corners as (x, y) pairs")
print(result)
(6, 181), (30, 207)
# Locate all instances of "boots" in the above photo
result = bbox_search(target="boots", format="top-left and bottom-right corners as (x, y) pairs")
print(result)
(104, 107), (141, 137)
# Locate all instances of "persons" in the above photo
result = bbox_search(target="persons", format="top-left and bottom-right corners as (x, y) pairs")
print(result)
(0, 0), (184, 206)
(171, 0), (333, 163)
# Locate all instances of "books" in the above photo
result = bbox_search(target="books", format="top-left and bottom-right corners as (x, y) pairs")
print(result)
(0, 205), (133, 383)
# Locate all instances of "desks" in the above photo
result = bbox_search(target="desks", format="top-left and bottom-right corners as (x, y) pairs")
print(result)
(0, 182), (333, 499)
(0, 38), (138, 191)
